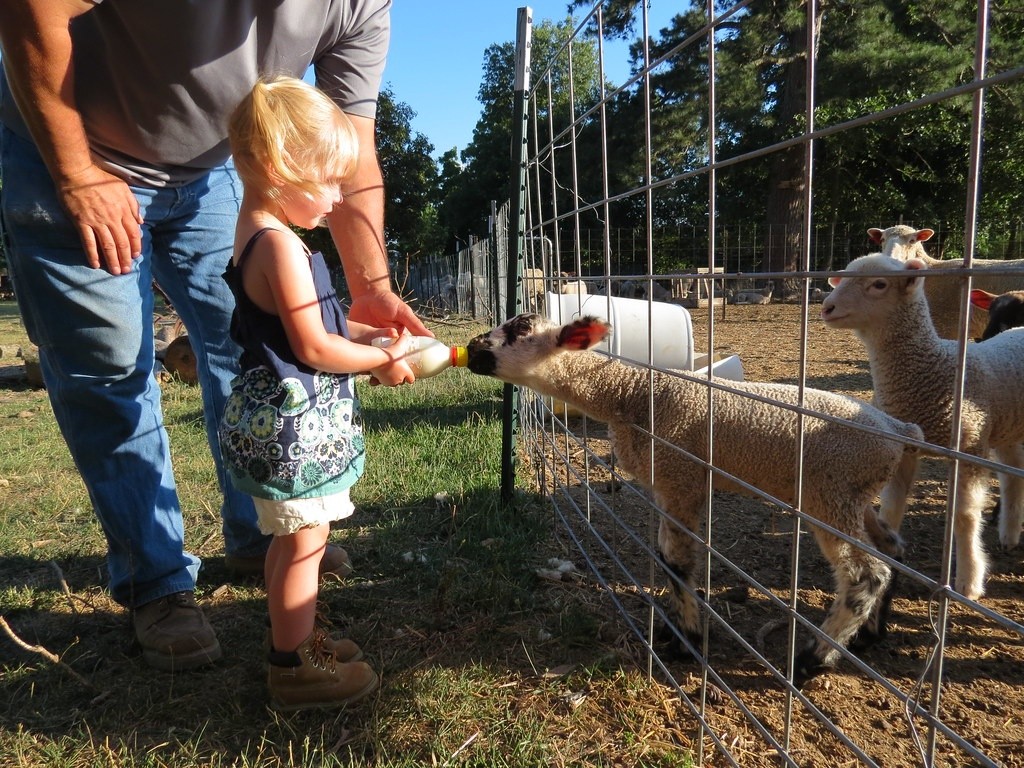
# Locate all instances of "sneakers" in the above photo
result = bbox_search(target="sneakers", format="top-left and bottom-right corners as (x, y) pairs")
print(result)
(225, 534), (352, 585)
(265, 611), (363, 664)
(134, 590), (221, 673)
(267, 632), (378, 712)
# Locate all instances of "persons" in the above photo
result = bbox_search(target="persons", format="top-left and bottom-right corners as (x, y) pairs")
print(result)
(224, 72), (415, 708)
(0, 0), (434, 665)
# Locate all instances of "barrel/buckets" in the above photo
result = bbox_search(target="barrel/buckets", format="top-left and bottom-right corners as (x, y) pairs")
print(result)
(543, 292), (695, 418)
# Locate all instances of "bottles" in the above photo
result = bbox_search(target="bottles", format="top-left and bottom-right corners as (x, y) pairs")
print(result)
(372, 336), (468, 382)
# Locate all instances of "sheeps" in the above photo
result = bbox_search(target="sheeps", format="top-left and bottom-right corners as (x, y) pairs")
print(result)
(552, 270), (588, 294)
(819, 251), (1024, 601)
(971, 288), (1023, 343)
(465, 311), (926, 695)
(732, 281), (775, 306)
(866, 225), (1023, 343)
